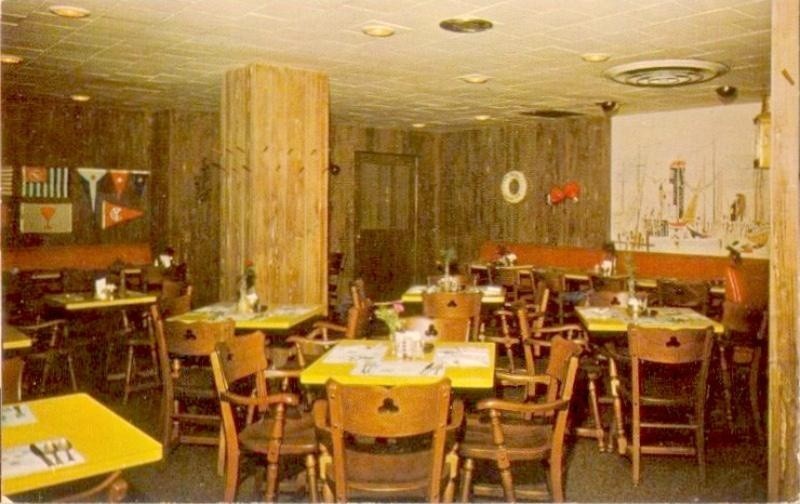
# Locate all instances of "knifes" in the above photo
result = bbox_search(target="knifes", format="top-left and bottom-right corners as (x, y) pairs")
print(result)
(29, 442), (55, 467)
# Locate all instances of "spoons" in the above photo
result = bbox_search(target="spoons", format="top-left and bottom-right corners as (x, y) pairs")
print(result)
(55, 438), (75, 463)
(43, 440), (65, 465)
